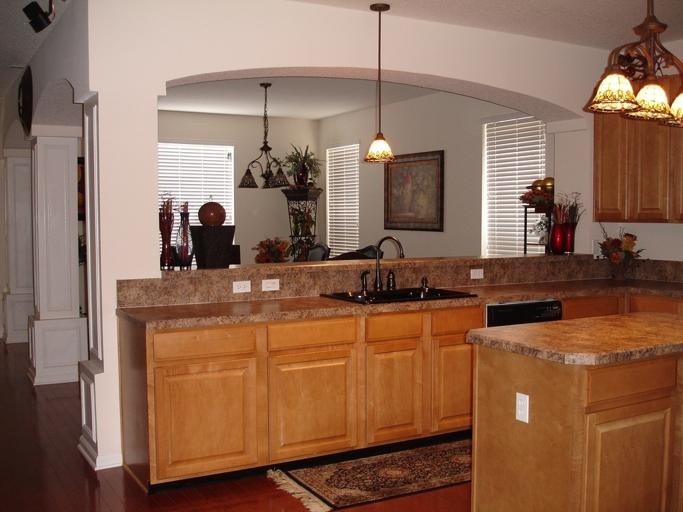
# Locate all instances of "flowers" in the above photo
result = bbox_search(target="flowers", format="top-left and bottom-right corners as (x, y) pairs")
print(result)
(250, 237), (290, 263)
(517, 190), (553, 204)
(592, 219), (649, 270)
(543, 191), (587, 224)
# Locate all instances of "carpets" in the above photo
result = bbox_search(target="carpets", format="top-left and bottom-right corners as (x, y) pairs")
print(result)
(265, 438), (470, 511)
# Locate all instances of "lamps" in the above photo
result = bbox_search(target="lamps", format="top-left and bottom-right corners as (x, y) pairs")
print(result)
(363, 3), (397, 164)
(21, 0), (58, 34)
(583, 1), (683, 129)
(237, 82), (290, 189)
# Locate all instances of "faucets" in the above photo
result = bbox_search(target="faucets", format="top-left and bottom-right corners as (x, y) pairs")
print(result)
(373, 235), (405, 292)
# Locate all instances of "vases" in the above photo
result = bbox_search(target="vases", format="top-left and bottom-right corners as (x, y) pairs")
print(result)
(174, 213), (195, 269)
(548, 223), (574, 254)
(612, 267), (625, 283)
(158, 212), (175, 270)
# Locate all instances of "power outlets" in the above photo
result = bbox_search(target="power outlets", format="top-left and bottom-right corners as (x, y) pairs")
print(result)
(230, 279), (252, 295)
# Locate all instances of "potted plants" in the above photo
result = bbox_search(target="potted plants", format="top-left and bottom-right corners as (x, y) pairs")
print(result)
(275, 142), (322, 188)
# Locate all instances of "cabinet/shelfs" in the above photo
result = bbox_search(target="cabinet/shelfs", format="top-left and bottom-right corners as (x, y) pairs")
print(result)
(471, 344), (683, 511)
(364, 306), (484, 446)
(561, 295), (618, 319)
(116, 314), (357, 492)
(522, 204), (551, 254)
(591, 73), (682, 225)
(280, 187), (324, 262)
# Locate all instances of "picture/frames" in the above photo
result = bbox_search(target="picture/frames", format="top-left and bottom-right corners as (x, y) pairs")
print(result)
(382, 149), (444, 232)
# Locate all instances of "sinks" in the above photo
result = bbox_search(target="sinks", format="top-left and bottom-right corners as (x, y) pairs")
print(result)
(318, 287), (478, 308)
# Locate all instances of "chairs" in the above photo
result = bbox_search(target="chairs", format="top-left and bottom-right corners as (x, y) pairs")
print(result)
(354, 244), (384, 259)
(329, 251), (367, 258)
(292, 242), (331, 261)
